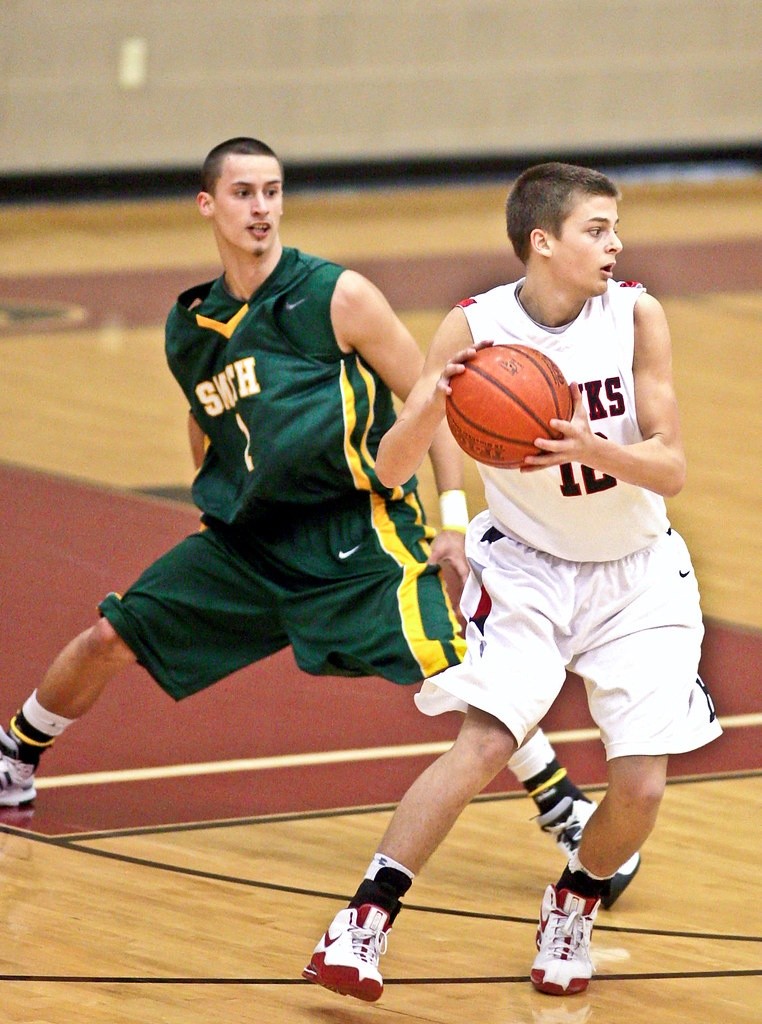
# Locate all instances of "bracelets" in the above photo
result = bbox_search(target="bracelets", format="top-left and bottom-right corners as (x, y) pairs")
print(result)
(438, 490), (471, 532)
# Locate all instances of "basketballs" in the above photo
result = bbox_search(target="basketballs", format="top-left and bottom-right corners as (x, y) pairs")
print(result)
(444, 343), (575, 471)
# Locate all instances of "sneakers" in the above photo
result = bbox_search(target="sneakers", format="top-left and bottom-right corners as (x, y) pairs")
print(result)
(301, 904), (393, 1002)
(529, 883), (603, 996)
(537, 795), (641, 909)
(0, 725), (37, 807)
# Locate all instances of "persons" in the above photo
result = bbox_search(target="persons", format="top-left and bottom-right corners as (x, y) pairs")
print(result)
(302, 162), (725, 1001)
(0, 135), (641, 911)
